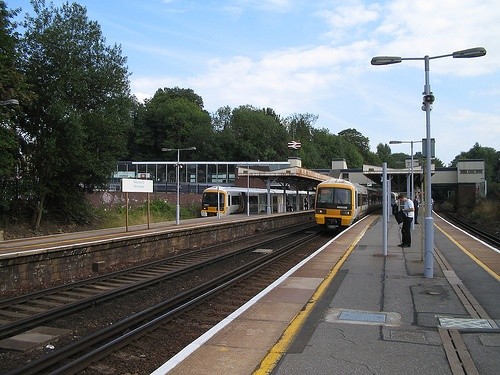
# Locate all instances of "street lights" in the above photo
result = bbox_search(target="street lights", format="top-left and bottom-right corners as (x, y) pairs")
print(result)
(371, 47), (487, 279)
(161, 147), (196, 225)
(389, 141), (422, 199)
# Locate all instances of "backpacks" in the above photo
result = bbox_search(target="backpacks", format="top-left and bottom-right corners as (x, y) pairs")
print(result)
(394, 210), (408, 225)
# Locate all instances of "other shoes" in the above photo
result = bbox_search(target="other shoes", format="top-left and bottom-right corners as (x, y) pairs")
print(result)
(414, 221), (419, 223)
(398, 242), (410, 247)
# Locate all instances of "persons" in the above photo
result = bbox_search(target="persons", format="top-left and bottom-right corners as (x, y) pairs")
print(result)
(391, 194), (420, 245)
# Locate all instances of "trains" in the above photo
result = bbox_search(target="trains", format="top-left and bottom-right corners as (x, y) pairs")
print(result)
(314, 178), (400, 232)
(201, 185), (316, 217)
